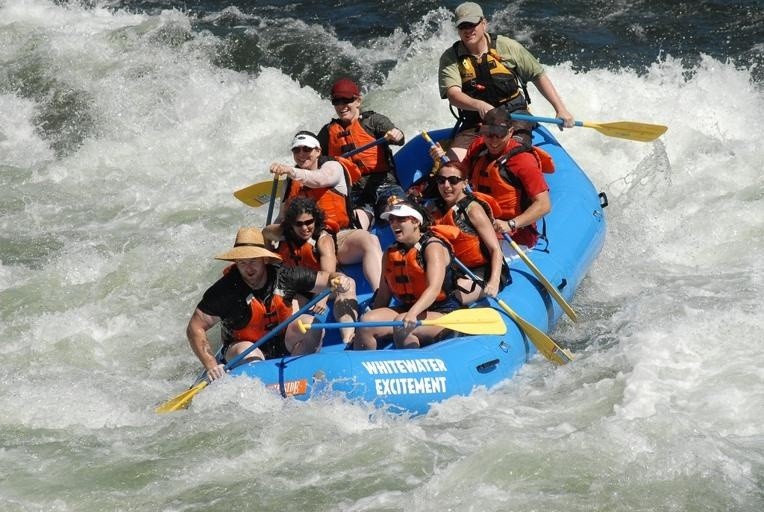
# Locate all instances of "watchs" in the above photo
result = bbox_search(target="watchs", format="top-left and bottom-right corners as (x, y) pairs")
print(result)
(507, 220), (517, 233)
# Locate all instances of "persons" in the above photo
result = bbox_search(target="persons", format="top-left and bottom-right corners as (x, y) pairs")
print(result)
(316, 78), (407, 232)
(353, 200), (466, 352)
(261, 195), (358, 345)
(185, 224), (351, 384)
(269, 131), (384, 295)
(428, 106), (551, 264)
(427, 160), (513, 306)
(437, 2), (576, 164)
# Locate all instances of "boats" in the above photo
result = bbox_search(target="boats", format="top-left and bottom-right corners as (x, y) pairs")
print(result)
(192, 120), (609, 419)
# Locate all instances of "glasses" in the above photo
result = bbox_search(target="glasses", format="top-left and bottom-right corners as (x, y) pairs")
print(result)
(389, 214), (416, 221)
(332, 98), (357, 105)
(292, 146), (313, 152)
(435, 174), (465, 184)
(293, 219), (314, 227)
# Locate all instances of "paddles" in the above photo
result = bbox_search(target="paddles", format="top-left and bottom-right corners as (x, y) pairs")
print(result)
(450, 253), (572, 366)
(151, 278), (340, 414)
(297, 307), (508, 335)
(456, 102), (668, 142)
(233, 130), (392, 207)
(421, 129), (578, 322)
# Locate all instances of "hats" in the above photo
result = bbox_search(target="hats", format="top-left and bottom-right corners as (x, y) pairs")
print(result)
(331, 80), (359, 99)
(380, 205), (424, 225)
(480, 107), (512, 137)
(215, 228), (282, 261)
(292, 135), (320, 148)
(454, 2), (484, 28)
